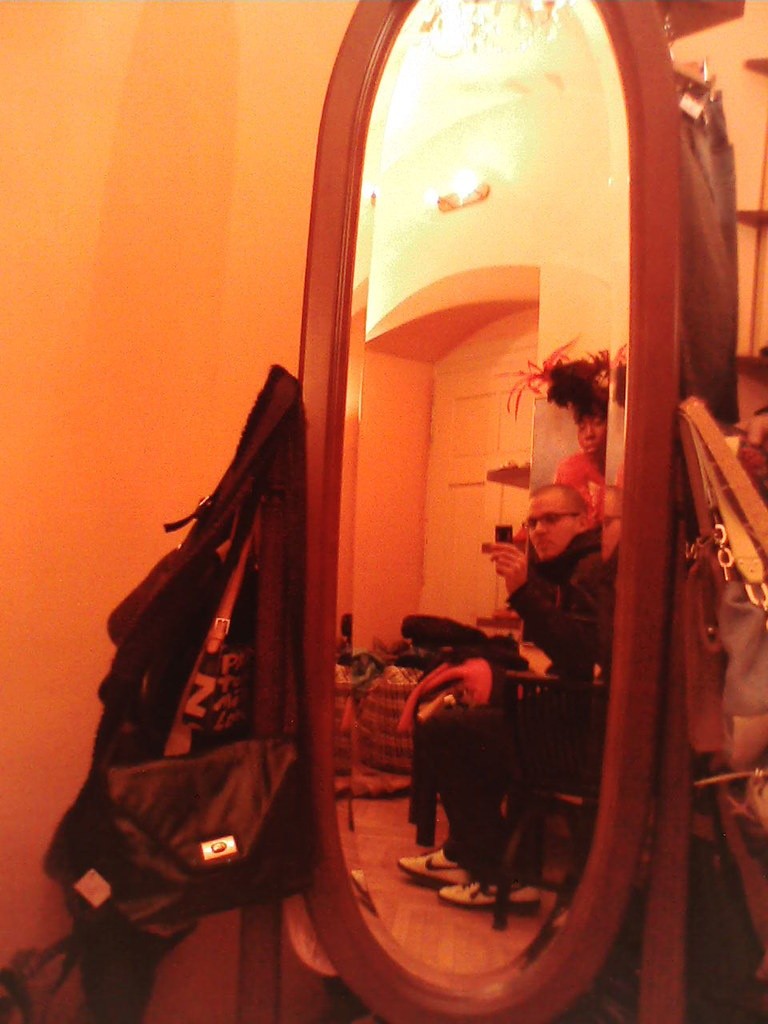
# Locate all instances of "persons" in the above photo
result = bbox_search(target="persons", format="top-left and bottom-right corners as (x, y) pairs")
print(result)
(397, 351), (627, 913)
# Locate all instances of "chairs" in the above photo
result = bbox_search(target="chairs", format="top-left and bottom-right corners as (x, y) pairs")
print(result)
(494, 670), (585, 928)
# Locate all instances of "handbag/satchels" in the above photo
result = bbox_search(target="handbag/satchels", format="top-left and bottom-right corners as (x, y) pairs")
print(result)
(677, 397), (768, 769)
(46, 363), (312, 940)
(353, 652), (418, 772)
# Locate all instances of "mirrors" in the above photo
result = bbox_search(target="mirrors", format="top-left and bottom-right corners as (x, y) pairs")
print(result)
(302, 1), (692, 1024)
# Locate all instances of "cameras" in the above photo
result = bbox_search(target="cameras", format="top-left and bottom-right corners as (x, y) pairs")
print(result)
(495, 525), (513, 544)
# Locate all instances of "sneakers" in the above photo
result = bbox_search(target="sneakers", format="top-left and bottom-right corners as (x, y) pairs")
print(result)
(398, 846), (475, 885)
(438, 875), (541, 914)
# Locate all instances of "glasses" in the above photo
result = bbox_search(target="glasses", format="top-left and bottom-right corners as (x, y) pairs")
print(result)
(523, 511), (579, 531)
